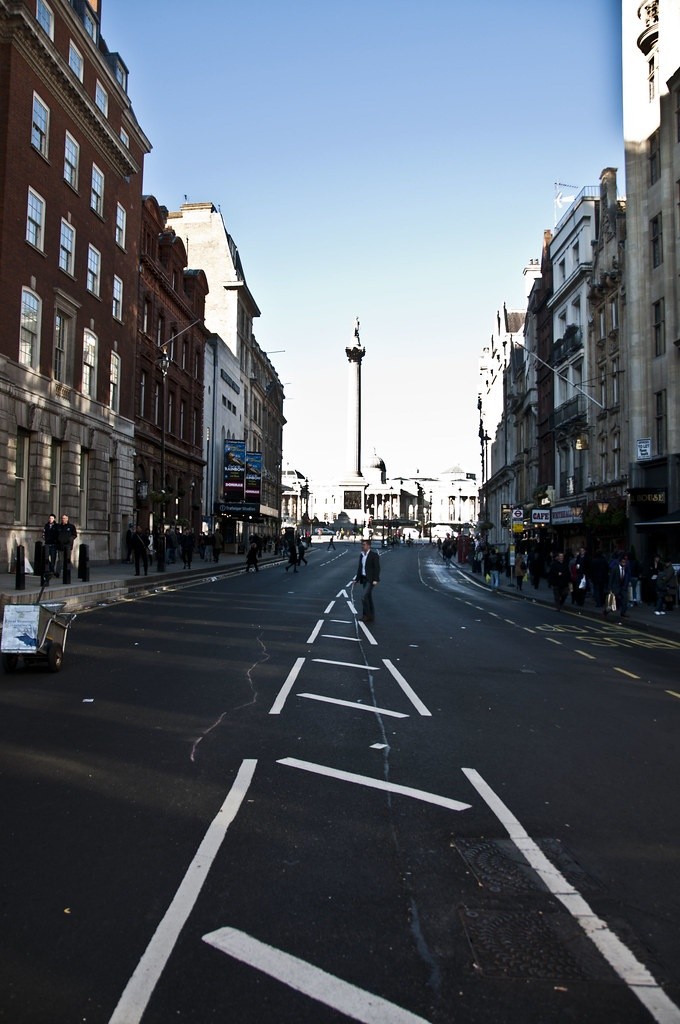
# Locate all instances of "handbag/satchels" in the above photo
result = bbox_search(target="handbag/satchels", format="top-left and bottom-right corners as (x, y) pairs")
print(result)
(578, 574), (586, 589)
(522, 573), (527, 582)
(486, 574), (491, 583)
(607, 593), (616, 612)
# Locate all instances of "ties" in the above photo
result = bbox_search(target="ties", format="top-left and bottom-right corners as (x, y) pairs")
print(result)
(621, 568), (624, 577)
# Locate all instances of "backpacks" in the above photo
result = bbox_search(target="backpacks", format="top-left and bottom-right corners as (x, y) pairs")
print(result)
(520, 562), (527, 570)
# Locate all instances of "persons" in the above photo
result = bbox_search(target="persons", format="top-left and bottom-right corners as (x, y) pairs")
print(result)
(43, 514), (58, 572)
(243, 533), (308, 574)
(326, 538), (336, 550)
(59, 514), (77, 574)
(442, 532), (680, 629)
(355, 540), (381, 622)
(127, 523), (223, 576)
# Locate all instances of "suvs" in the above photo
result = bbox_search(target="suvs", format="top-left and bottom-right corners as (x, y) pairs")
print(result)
(315, 528), (335, 535)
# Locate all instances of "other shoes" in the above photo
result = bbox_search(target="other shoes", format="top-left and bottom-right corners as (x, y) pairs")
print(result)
(54, 572), (59, 578)
(183, 565), (191, 569)
(286, 567), (298, 573)
(360, 616), (374, 622)
(517, 586), (522, 591)
(305, 563), (307, 565)
(654, 611), (666, 616)
(135, 572), (147, 576)
(621, 613), (629, 617)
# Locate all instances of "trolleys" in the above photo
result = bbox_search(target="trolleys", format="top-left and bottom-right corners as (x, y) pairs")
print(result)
(0, 601), (76, 675)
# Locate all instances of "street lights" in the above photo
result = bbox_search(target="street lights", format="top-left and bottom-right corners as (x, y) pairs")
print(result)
(156, 345), (170, 573)
